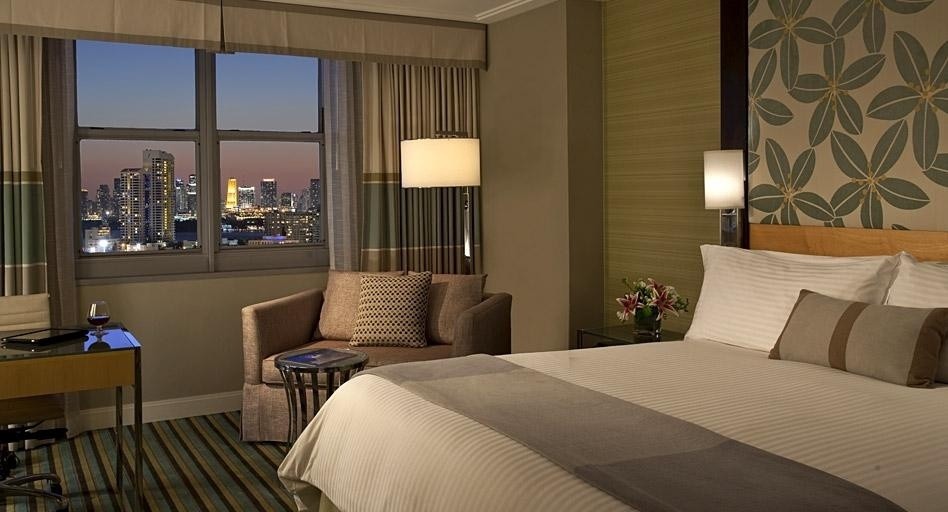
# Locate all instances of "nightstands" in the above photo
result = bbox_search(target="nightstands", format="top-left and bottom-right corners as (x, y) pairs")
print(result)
(577, 324), (685, 347)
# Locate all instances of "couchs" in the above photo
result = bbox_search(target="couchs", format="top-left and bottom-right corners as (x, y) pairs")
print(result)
(238, 287), (515, 447)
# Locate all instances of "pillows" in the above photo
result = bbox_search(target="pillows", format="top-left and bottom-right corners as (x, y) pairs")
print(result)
(767, 286), (947, 387)
(408, 269), (488, 346)
(885, 251), (948, 309)
(344, 272), (433, 347)
(313, 269), (405, 342)
(686, 241), (898, 358)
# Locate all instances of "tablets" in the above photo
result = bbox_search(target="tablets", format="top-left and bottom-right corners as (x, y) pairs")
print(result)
(3, 328), (88, 345)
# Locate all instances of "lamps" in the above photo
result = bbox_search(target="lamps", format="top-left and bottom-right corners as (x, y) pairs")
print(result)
(396, 133), (481, 272)
(702, 148), (745, 220)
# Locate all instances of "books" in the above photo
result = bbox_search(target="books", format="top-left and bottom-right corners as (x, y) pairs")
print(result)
(282, 348), (357, 366)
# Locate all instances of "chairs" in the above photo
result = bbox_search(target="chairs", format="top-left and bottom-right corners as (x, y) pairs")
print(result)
(0, 293), (70, 508)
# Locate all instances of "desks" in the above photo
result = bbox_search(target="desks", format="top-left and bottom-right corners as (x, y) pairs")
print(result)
(274, 346), (370, 455)
(0, 319), (145, 510)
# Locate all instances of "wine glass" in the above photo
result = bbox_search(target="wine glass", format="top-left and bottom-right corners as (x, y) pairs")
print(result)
(86, 336), (111, 354)
(87, 300), (111, 336)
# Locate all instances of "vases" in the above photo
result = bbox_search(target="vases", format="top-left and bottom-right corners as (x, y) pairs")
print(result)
(633, 312), (664, 343)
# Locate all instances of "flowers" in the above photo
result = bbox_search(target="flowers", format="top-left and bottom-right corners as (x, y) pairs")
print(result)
(611, 275), (692, 324)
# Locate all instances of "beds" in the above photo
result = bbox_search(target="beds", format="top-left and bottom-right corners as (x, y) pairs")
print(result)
(275, 224), (948, 512)
